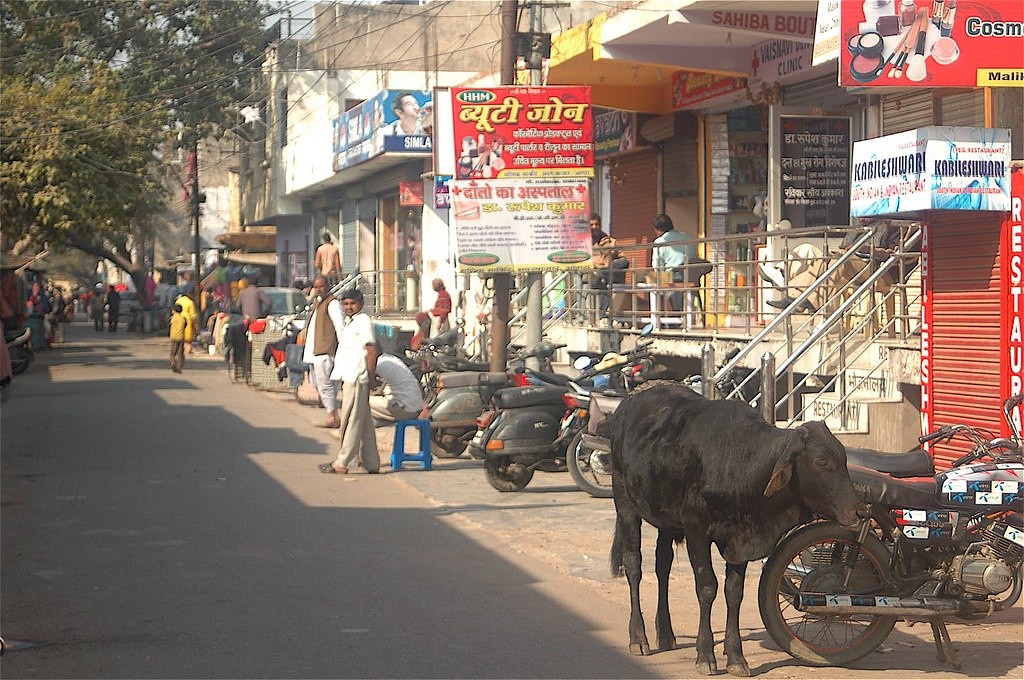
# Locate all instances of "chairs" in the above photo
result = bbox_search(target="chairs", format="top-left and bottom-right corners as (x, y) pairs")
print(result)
(673, 258), (714, 328)
(845, 255), (919, 339)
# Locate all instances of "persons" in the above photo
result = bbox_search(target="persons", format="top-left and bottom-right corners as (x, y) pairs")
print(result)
(316, 232), (341, 288)
(410, 278), (452, 350)
(154, 278), (170, 307)
(92, 285), (121, 332)
(318, 289), (379, 474)
(169, 276), (271, 373)
(301, 274), (349, 429)
(24, 277), (94, 351)
(368, 339), (423, 430)
(364, 92), (433, 137)
(757, 218), (922, 337)
(650, 213), (697, 326)
(0, 320), (12, 387)
(581, 211), (630, 320)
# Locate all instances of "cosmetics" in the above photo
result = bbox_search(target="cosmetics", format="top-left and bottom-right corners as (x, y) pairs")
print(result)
(493, 157), (505, 170)
(848, 0), (918, 83)
(456, 133), (485, 177)
(930, 37), (960, 66)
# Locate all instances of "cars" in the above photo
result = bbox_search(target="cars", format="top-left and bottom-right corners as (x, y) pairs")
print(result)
(114, 290), (138, 324)
(232, 287), (307, 320)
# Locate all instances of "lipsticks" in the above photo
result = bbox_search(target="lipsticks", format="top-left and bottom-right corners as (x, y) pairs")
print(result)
(492, 137), (503, 155)
(931, 0), (956, 37)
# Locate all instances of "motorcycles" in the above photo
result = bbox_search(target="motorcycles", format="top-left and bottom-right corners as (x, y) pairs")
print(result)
(757, 393), (1024, 672)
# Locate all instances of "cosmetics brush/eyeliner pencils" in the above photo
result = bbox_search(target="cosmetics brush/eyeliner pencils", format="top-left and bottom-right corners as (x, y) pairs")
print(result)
(875, 7), (929, 82)
(467, 145), (490, 177)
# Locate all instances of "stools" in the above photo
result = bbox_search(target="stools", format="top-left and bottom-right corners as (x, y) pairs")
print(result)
(391, 418), (432, 471)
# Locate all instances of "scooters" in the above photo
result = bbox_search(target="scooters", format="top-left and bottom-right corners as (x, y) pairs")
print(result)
(3, 326), (32, 376)
(401, 314), (747, 498)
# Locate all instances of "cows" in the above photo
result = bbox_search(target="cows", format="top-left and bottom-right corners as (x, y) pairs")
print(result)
(610, 384), (867, 677)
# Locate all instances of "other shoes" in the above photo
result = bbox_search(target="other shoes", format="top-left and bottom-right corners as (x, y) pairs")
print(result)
(319, 462), (346, 475)
(313, 418), (342, 428)
